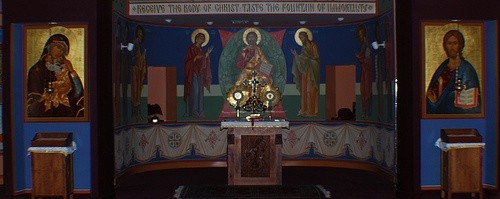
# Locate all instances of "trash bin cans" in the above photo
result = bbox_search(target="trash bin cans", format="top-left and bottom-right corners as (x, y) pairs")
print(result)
(26, 132), (75, 199)
(435, 127), (487, 198)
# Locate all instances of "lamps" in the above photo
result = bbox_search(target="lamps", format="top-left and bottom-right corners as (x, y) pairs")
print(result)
(371, 39), (387, 50)
(120, 41), (135, 53)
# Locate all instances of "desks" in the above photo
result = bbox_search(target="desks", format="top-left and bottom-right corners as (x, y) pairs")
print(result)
(220, 121), (289, 186)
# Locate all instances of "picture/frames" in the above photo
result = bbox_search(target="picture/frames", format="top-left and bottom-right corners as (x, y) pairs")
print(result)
(20, 24), (90, 123)
(418, 20), (487, 120)
